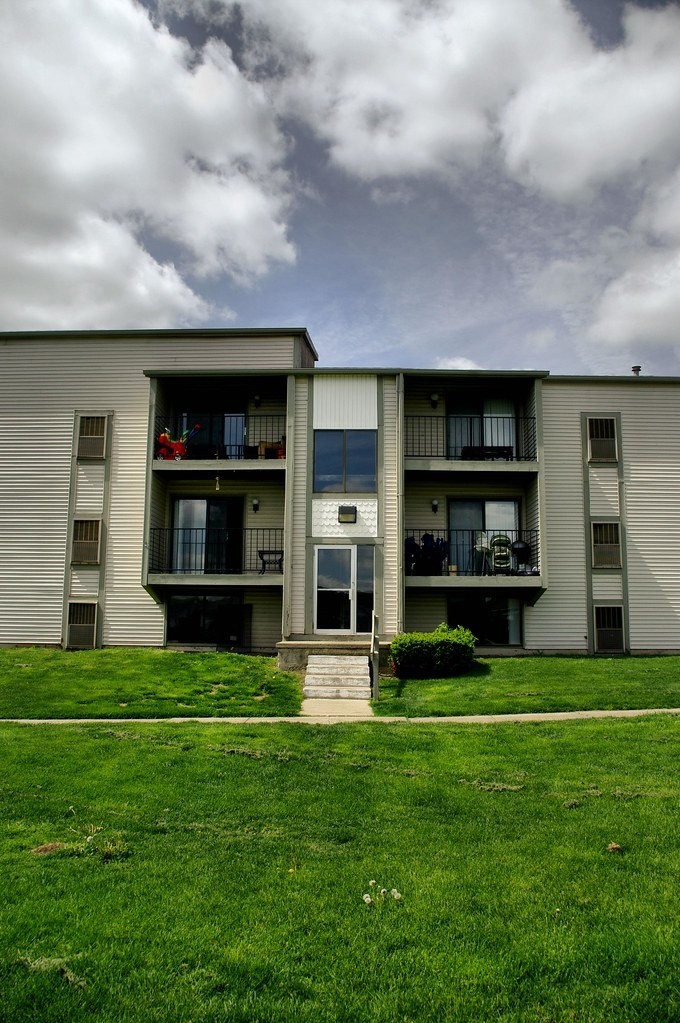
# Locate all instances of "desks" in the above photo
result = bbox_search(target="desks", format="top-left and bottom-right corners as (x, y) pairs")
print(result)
(461, 446), (514, 461)
(243, 445), (259, 459)
(258, 550), (284, 575)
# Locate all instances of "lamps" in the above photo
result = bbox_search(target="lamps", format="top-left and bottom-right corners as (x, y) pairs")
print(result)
(431, 394), (439, 410)
(215, 477), (220, 490)
(432, 499), (439, 514)
(252, 498), (259, 514)
(253, 395), (260, 409)
(338, 505), (357, 523)
